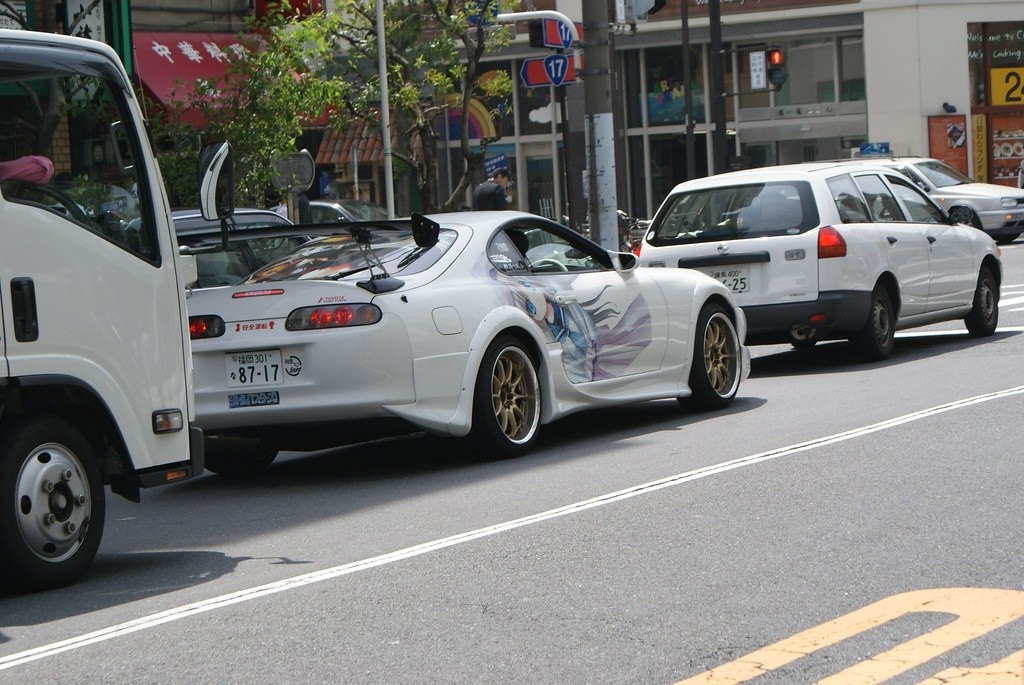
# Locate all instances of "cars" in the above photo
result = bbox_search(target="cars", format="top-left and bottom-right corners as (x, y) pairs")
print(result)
(634, 160), (1003, 365)
(784, 154), (1023, 245)
(171, 204), (314, 292)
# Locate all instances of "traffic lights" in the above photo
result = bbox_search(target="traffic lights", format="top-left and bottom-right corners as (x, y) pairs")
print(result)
(769, 48), (790, 88)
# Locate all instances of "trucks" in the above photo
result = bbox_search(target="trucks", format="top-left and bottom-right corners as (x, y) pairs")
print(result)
(0, 32), (239, 590)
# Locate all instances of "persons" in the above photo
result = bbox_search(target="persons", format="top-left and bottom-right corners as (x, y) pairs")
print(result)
(473, 167), (511, 212)
(505, 229), (529, 259)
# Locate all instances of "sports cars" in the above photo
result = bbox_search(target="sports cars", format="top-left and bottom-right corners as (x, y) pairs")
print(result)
(180, 209), (752, 465)
